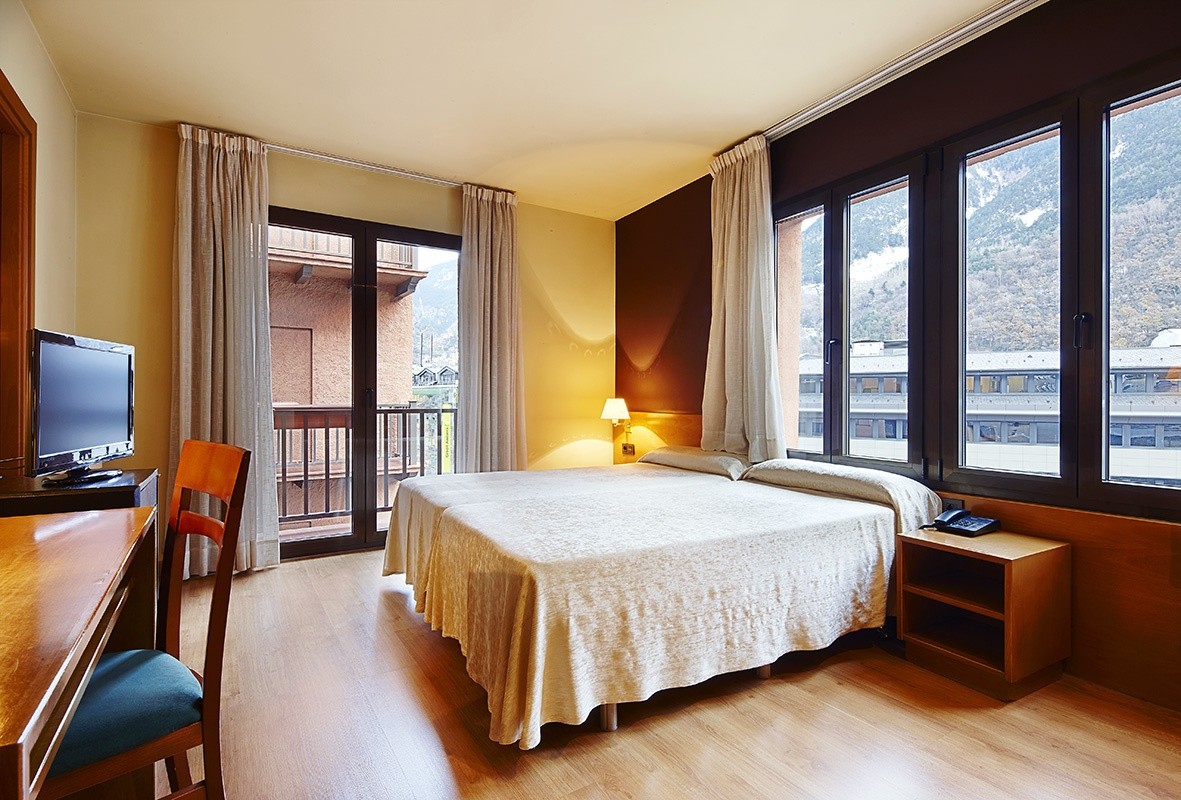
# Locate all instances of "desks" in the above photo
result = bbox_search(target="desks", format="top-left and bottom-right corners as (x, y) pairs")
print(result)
(0, 504), (159, 800)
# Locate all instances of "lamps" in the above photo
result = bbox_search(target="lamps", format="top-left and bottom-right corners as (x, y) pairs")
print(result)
(599, 397), (631, 434)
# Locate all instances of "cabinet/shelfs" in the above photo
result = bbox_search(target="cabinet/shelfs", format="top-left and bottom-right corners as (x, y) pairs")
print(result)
(0, 467), (160, 608)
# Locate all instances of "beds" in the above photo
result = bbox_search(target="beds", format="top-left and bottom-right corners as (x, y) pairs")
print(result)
(384, 444), (945, 753)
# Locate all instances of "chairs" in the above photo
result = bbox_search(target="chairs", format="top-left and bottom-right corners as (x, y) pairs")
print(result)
(37, 440), (252, 800)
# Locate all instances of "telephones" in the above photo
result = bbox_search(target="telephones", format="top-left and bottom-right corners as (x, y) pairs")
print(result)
(934, 508), (1001, 537)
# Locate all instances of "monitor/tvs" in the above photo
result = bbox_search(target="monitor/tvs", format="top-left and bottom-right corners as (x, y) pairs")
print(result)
(26, 328), (135, 489)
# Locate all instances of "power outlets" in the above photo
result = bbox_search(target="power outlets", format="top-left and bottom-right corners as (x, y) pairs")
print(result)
(942, 498), (964, 512)
(622, 444), (635, 456)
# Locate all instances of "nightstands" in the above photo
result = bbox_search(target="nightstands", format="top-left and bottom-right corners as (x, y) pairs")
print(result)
(896, 526), (1074, 703)
